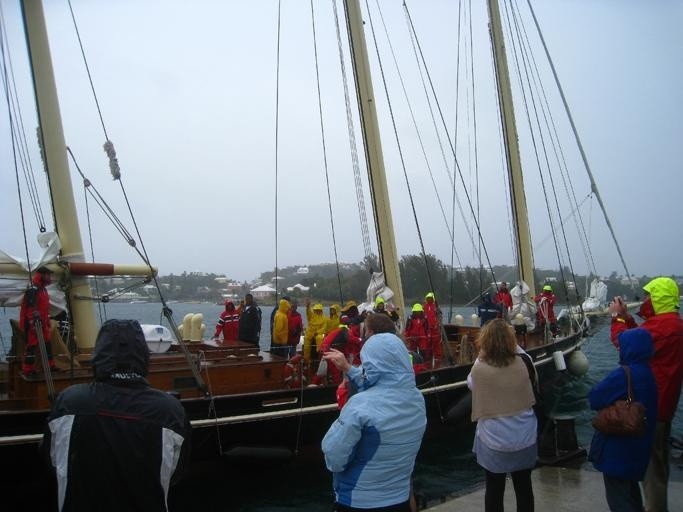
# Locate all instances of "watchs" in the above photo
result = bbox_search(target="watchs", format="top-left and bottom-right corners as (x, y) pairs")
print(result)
(609, 310), (622, 319)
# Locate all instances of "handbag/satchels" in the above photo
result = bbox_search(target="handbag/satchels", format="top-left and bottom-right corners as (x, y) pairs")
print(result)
(592, 366), (648, 434)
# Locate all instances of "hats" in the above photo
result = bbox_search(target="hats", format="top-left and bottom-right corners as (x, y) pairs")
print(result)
(36, 266), (54, 273)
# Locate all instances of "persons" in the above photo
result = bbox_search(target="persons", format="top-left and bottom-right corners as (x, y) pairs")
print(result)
(319, 333), (428, 512)
(477, 291), (503, 328)
(494, 282), (513, 315)
(467, 318), (539, 510)
(37, 318), (190, 509)
(16, 266), (62, 381)
(533, 285), (561, 336)
(210, 292), (444, 387)
(586, 328), (661, 512)
(606, 277), (683, 511)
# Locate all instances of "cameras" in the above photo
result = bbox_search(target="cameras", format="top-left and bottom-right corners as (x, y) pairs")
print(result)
(608, 298), (623, 306)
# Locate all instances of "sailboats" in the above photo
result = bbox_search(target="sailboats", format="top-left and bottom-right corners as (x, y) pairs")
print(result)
(0, 0), (645, 480)
